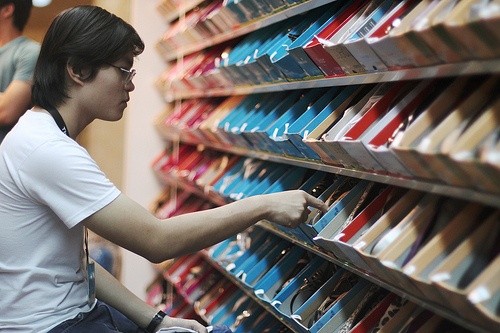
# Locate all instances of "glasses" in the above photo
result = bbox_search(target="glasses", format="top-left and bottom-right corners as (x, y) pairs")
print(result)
(103, 61), (137, 83)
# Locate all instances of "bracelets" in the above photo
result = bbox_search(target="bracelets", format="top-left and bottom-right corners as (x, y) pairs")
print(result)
(147, 310), (167, 333)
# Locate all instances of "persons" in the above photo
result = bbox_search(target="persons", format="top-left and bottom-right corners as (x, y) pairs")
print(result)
(0, 5), (330, 333)
(0, 0), (44, 141)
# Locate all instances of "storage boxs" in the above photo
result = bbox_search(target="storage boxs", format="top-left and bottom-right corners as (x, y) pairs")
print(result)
(144, 0), (500, 333)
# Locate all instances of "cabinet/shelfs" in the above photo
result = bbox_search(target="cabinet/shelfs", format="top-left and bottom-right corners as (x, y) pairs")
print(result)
(142, 0), (500, 333)
